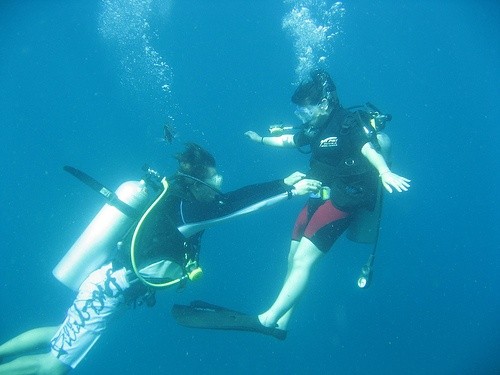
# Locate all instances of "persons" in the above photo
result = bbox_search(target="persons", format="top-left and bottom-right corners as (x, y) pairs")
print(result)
(1, 143), (323, 375)
(172, 69), (411, 339)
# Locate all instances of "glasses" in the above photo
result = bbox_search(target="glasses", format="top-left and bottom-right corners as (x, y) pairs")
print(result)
(294, 108), (314, 124)
(205, 174), (223, 186)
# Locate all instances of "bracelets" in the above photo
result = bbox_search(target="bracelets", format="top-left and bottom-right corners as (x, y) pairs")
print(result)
(262, 137), (263, 143)
